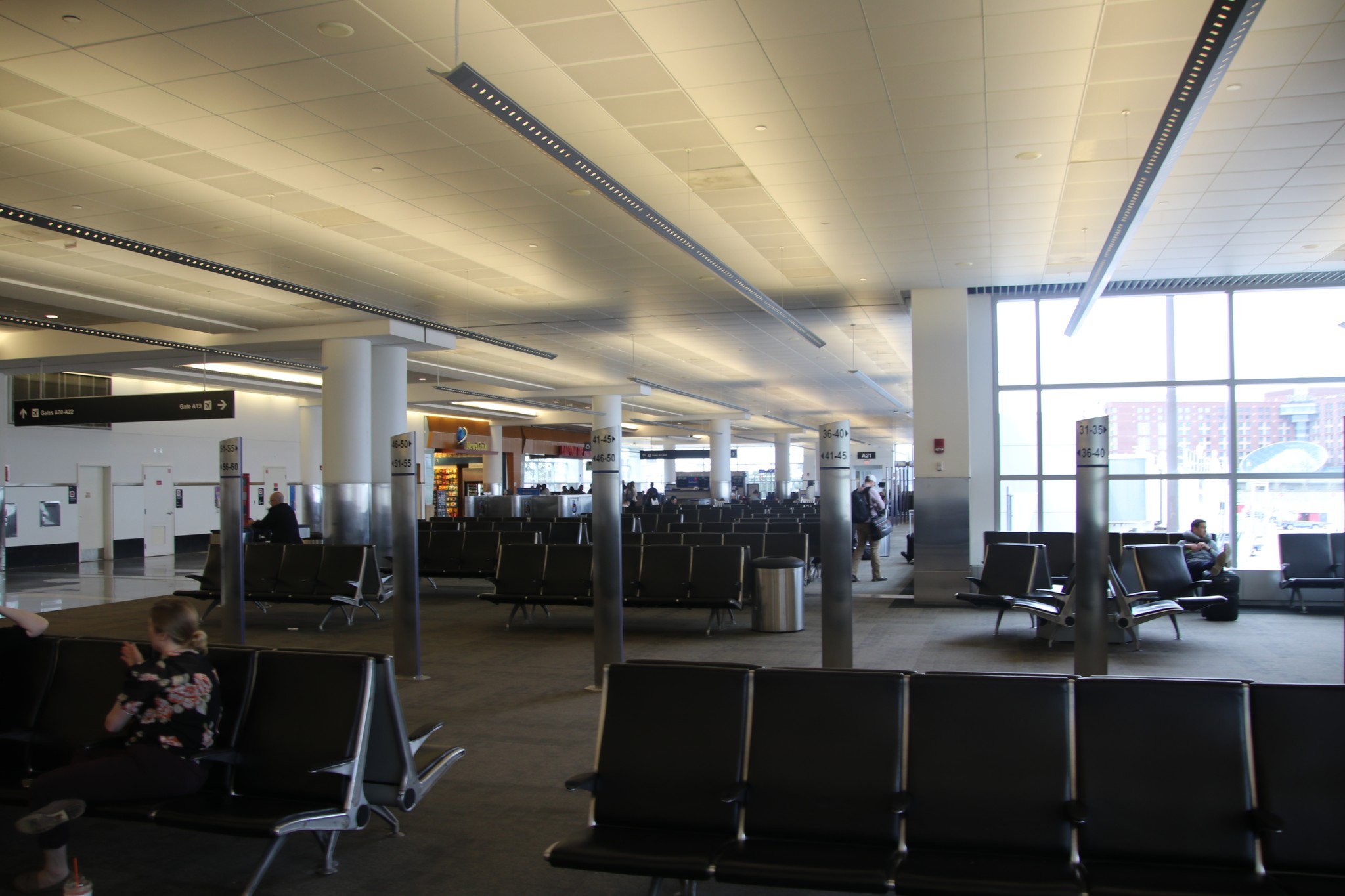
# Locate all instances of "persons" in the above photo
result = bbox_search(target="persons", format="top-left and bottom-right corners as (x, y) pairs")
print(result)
(1176, 519), (1231, 580)
(852, 473), (888, 582)
(531, 483), (592, 495)
(11, 597), (225, 893)
(247, 492), (305, 545)
(720, 489), (782, 506)
(622, 480), (680, 507)
(0, 604), (51, 651)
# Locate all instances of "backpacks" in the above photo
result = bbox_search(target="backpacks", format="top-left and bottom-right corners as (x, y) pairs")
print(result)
(852, 486), (872, 524)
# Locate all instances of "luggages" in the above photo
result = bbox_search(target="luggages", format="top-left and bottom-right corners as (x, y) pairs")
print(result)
(1201, 569), (1241, 621)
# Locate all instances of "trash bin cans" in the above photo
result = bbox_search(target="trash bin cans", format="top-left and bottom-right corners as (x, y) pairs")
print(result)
(751, 555), (806, 633)
(868, 535), (890, 556)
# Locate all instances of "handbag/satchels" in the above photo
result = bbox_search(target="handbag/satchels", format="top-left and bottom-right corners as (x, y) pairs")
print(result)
(871, 513), (894, 540)
(861, 545), (872, 560)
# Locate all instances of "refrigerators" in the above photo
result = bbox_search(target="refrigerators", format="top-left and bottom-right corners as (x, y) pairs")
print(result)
(464, 481), (483, 496)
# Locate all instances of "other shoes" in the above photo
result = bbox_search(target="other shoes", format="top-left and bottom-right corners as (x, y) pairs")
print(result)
(15, 798), (85, 835)
(12, 871), (74, 896)
(901, 551), (907, 558)
(1211, 552), (1228, 577)
(872, 578), (888, 581)
(852, 575), (861, 582)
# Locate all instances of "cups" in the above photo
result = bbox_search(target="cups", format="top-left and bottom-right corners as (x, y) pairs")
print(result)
(64, 875), (93, 896)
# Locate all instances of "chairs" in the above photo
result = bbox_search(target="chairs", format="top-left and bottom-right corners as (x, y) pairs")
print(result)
(173, 538), (397, 621)
(953, 528), (1345, 648)
(417, 500), (821, 630)
(0, 635), (467, 896)
(545, 659), (1345, 896)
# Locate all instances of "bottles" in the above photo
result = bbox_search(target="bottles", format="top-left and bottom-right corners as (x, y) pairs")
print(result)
(469, 483), (478, 494)
